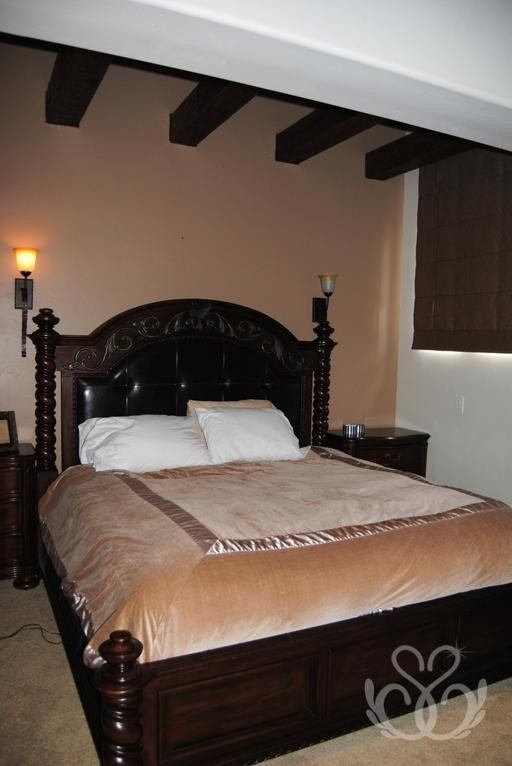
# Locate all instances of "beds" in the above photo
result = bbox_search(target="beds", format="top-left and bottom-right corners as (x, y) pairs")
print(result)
(32, 308), (512, 766)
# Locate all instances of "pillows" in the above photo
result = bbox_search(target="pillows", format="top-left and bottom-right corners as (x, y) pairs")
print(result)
(77, 399), (314, 473)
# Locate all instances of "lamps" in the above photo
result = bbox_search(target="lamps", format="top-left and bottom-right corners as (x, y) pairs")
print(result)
(311, 272), (339, 323)
(12, 247), (34, 358)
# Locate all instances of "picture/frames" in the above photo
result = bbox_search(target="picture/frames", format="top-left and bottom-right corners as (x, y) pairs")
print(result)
(0, 410), (19, 453)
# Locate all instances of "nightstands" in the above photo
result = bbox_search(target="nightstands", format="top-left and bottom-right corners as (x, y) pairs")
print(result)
(0, 443), (35, 582)
(325, 425), (430, 479)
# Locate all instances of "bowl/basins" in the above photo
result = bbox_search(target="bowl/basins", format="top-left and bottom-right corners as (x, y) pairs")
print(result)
(344, 423), (367, 439)
